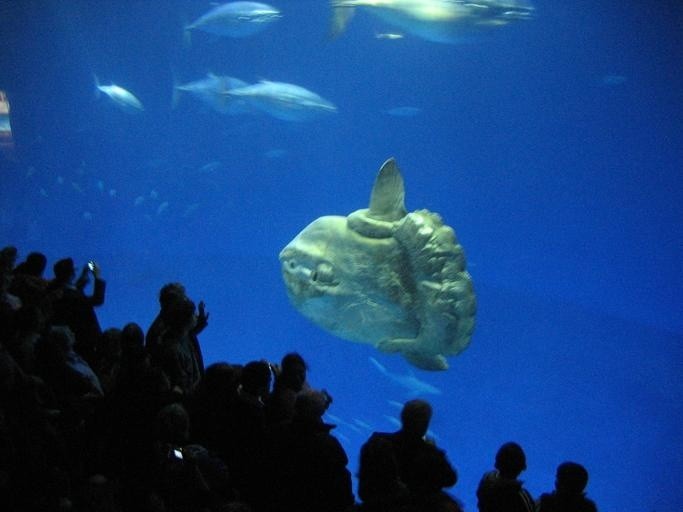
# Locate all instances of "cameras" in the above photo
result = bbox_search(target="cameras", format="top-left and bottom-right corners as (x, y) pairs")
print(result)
(88, 261), (95, 271)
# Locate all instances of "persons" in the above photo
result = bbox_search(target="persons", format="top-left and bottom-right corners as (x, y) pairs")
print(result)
(1, 247), (599, 512)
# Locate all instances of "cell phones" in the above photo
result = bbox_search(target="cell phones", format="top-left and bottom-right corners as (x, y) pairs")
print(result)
(173, 448), (183, 460)
